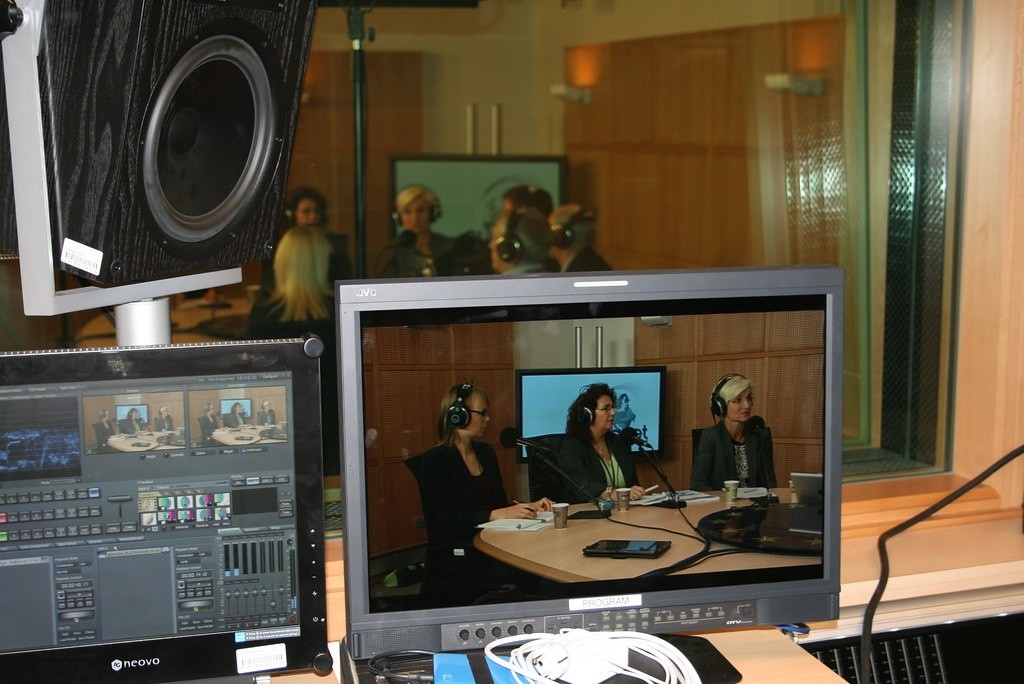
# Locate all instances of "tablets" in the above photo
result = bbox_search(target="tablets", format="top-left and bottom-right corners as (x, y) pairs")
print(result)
(585, 539), (673, 556)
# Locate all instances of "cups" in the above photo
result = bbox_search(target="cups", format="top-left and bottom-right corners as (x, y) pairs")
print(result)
(551, 503), (569, 530)
(724, 480), (739, 503)
(264, 423), (268, 429)
(124, 434), (128, 439)
(245, 285), (261, 304)
(161, 429), (166, 434)
(616, 488), (631, 512)
(227, 428), (232, 434)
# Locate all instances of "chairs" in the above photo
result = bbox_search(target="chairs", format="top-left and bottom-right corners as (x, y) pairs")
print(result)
(402, 454), (440, 608)
(691, 426), (773, 467)
(526, 434), (566, 502)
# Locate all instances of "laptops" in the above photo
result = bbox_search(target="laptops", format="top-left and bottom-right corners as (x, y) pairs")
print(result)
(790, 473), (824, 535)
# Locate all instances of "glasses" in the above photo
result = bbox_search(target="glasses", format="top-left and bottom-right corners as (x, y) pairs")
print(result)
(595, 404), (616, 411)
(471, 409), (487, 417)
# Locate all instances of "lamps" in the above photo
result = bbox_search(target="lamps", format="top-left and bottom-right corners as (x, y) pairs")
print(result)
(549, 84), (594, 104)
(639, 317), (671, 328)
(763, 71), (824, 96)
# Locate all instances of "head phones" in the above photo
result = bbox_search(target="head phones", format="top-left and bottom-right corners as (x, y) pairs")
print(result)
(283, 207), (295, 228)
(711, 373), (746, 418)
(158, 406), (163, 416)
(575, 385), (593, 426)
(261, 400), (266, 409)
(552, 206), (597, 249)
(495, 212), (525, 263)
(447, 377), (475, 428)
(391, 185), (442, 226)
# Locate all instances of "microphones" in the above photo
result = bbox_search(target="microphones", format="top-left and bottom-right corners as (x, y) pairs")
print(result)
(498, 427), (552, 454)
(382, 230), (415, 252)
(619, 427), (653, 450)
(747, 415), (765, 440)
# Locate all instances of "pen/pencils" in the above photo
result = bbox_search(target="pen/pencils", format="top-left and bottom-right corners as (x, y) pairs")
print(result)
(639, 483), (660, 492)
(511, 499), (535, 514)
(745, 487), (759, 493)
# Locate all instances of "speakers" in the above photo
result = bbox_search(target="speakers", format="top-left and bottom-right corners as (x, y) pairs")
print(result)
(37, 0), (316, 288)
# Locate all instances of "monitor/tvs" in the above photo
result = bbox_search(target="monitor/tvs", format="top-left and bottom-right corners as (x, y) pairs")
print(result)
(219, 399), (253, 419)
(335, 264), (841, 660)
(0, 332), (332, 684)
(515, 365), (667, 463)
(115, 404), (149, 424)
(389, 152), (566, 243)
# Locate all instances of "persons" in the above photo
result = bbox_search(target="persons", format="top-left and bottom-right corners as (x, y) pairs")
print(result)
(690, 374), (779, 488)
(415, 379), (556, 605)
(95, 407), (184, 452)
(246, 183), (615, 339)
(547, 384), (647, 502)
(202, 400), (274, 446)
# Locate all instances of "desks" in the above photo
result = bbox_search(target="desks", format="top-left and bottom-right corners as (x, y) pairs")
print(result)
(269, 627), (850, 684)
(107, 431), (186, 452)
(78, 297), (255, 348)
(473, 487), (822, 583)
(211, 426), (287, 445)
